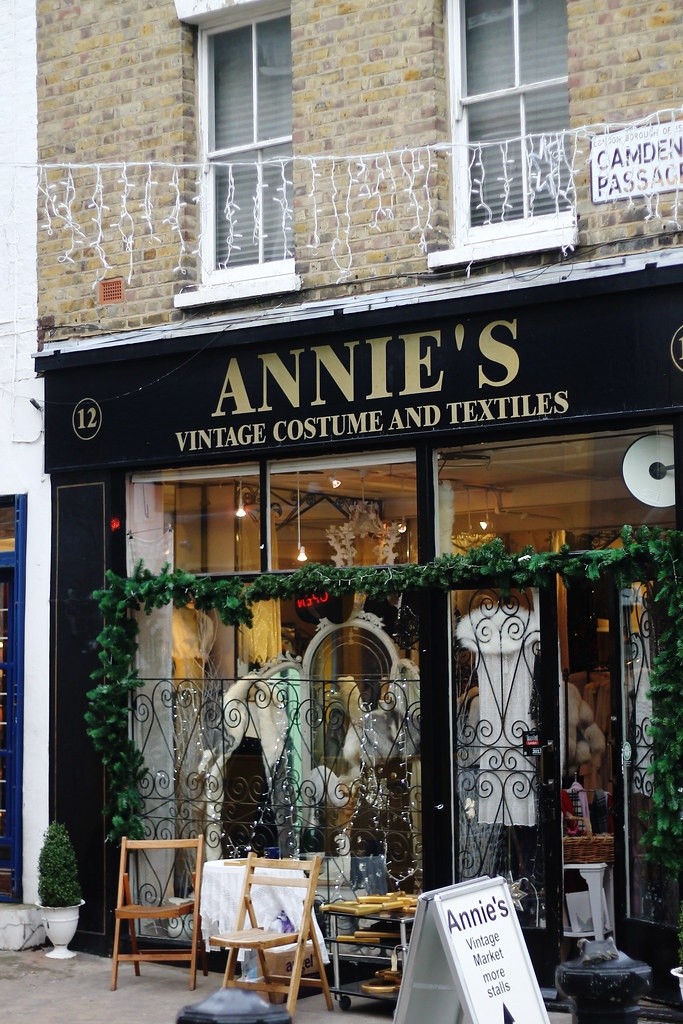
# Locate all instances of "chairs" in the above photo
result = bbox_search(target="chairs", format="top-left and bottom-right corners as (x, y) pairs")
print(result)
(111, 835), (209, 992)
(209, 852), (334, 1020)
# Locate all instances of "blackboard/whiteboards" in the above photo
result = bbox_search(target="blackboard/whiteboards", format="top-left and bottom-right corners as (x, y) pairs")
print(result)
(390, 875), (556, 1024)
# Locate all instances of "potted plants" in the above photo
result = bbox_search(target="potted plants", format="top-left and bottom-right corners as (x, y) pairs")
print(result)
(35, 820), (86, 959)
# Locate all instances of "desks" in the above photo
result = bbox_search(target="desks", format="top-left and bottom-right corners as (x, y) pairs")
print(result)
(198, 858), (331, 1002)
(564, 861), (612, 940)
(324, 909), (415, 1011)
